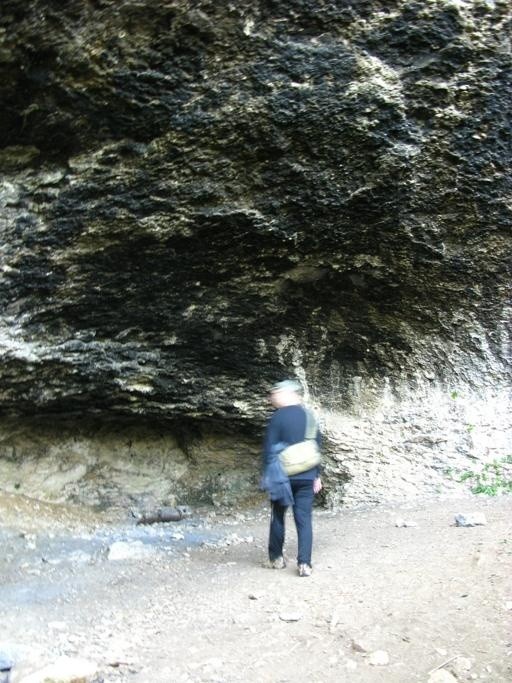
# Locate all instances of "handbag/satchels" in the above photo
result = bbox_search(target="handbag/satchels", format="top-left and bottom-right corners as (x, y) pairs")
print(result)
(278, 439), (323, 477)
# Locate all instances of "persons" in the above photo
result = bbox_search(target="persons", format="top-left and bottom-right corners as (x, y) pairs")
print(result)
(261, 380), (322, 577)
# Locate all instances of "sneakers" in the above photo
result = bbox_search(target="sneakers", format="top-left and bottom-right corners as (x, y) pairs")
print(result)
(298, 562), (312, 575)
(260, 557), (286, 570)
